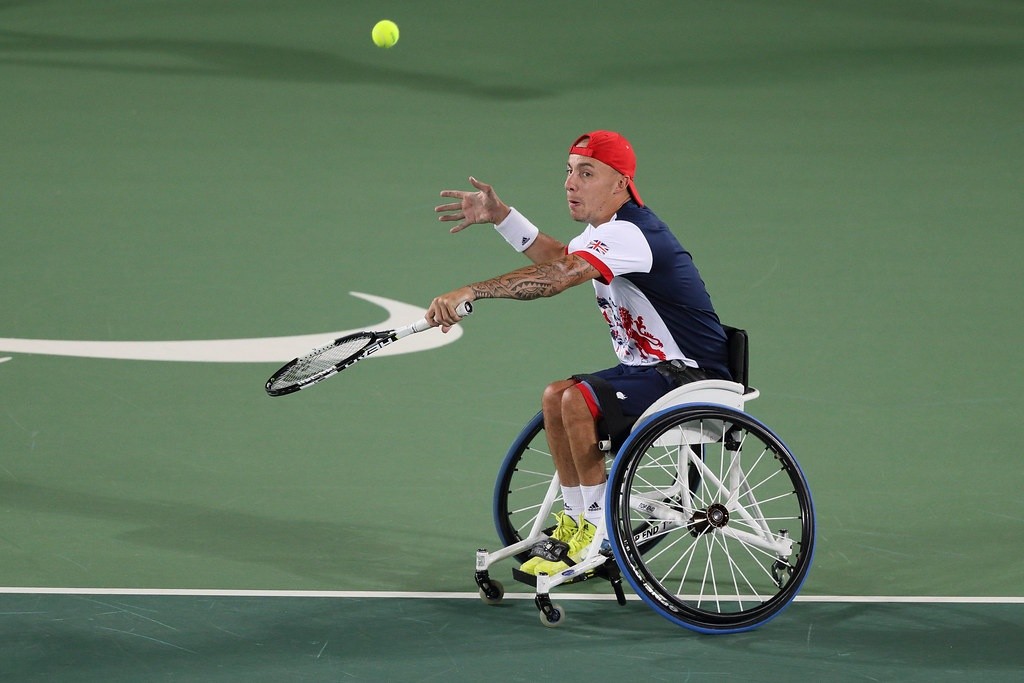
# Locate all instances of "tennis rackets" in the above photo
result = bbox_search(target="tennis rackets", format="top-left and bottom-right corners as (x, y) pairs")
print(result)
(265, 300), (475, 398)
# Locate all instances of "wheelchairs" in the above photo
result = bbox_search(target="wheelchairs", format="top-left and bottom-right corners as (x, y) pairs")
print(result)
(473, 322), (820, 635)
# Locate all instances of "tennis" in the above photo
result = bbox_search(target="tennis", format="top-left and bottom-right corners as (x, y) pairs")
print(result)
(371, 20), (401, 52)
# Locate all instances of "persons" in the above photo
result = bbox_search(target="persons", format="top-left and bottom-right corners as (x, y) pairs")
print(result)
(424, 131), (734, 581)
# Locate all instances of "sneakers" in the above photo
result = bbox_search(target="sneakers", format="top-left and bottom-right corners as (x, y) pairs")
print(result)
(534, 512), (597, 578)
(519, 509), (593, 583)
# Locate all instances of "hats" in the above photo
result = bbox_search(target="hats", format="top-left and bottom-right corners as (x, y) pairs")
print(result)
(567, 130), (645, 208)
